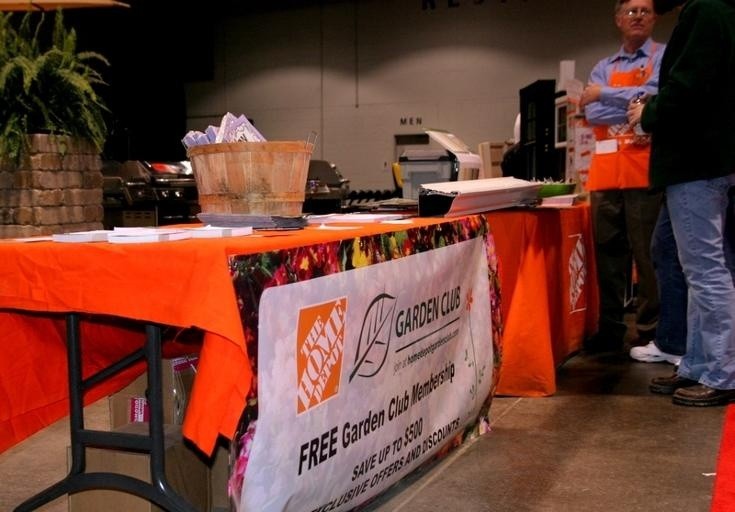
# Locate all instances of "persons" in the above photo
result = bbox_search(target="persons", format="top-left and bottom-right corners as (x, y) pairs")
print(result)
(580, 1), (669, 353)
(626, 0), (735, 406)
(629, 186), (690, 366)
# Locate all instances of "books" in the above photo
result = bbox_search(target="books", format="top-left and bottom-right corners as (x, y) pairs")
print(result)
(180, 111), (268, 150)
(49, 222), (254, 244)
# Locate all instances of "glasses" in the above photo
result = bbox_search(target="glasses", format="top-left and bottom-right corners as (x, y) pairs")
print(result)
(619, 6), (649, 18)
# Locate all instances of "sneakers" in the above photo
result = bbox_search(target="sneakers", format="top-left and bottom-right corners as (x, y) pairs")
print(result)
(631, 341), (683, 367)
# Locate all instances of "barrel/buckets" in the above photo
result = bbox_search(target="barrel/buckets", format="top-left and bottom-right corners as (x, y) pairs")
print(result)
(183, 129), (319, 219)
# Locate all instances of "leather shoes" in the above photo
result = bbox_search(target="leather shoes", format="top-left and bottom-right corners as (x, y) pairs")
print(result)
(673, 383), (734, 407)
(648, 373), (699, 395)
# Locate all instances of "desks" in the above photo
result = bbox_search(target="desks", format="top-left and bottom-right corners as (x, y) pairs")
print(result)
(344, 202), (596, 396)
(0, 212), (503, 511)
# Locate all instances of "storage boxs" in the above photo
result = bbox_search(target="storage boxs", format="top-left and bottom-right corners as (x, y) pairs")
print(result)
(66, 352), (236, 511)
